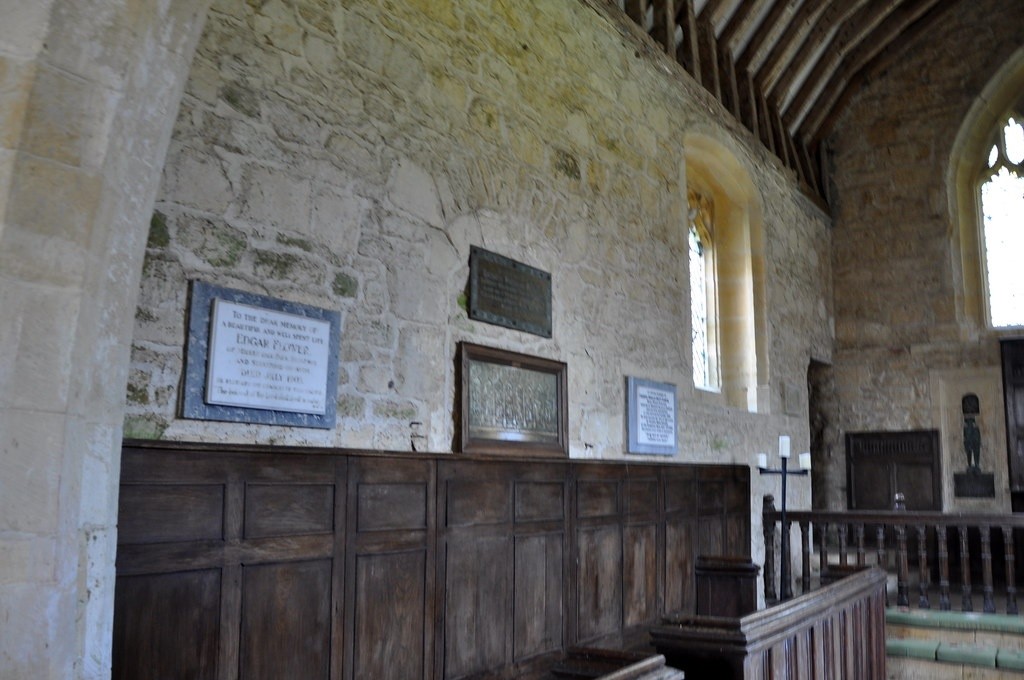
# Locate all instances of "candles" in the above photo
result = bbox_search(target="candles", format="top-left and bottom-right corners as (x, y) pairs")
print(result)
(778, 436), (791, 457)
(799, 453), (811, 470)
(758, 452), (767, 470)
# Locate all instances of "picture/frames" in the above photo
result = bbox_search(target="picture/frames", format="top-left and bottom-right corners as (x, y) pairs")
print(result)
(459, 340), (571, 459)
